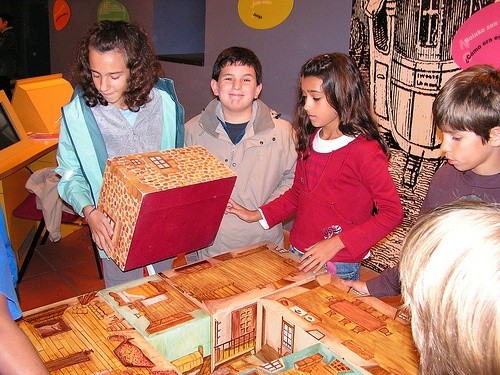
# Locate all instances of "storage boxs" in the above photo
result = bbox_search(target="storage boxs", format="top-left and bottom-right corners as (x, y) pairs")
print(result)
(96, 145), (238, 272)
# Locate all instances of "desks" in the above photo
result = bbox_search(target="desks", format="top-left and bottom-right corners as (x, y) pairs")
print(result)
(15, 239), (421, 375)
(0, 137), (58, 181)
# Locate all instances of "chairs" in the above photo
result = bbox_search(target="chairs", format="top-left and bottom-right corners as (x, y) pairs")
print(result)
(11, 193), (103, 283)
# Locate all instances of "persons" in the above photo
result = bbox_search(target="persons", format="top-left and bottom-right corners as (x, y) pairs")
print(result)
(55, 19), (200, 290)
(0, 205), (51, 375)
(182, 46), (296, 264)
(340, 64), (500, 300)
(0, 13), (18, 102)
(224, 53), (405, 284)
(399, 200), (500, 375)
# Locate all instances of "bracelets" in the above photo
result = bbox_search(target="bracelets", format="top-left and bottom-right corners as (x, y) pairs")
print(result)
(85, 209), (95, 222)
